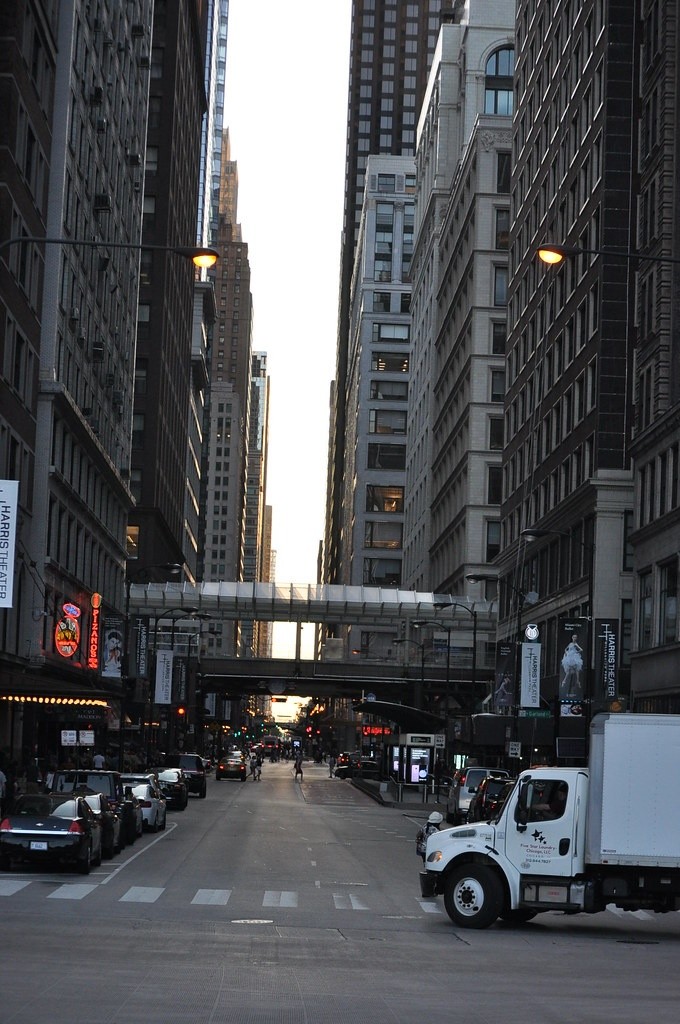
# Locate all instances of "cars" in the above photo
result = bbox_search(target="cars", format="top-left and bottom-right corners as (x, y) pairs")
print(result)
(201, 757), (211, 769)
(216, 750), (247, 782)
(117, 781), (167, 831)
(121, 771), (166, 809)
(115, 786), (143, 839)
(251, 746), (258, 752)
(334, 761), (379, 779)
(147, 767), (190, 811)
(488, 782), (551, 822)
(79, 793), (125, 860)
(0, 793), (105, 873)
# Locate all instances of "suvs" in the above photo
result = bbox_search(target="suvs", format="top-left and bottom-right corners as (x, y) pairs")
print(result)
(336, 753), (352, 766)
(54, 768), (132, 842)
(466, 774), (515, 827)
(162, 751), (206, 798)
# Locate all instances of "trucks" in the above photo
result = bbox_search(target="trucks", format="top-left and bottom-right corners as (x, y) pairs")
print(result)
(418, 710), (680, 927)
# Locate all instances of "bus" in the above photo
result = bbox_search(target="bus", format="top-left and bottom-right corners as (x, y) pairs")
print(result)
(262, 736), (279, 755)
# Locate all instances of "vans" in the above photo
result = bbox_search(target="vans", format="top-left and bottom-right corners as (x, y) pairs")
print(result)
(443, 765), (511, 826)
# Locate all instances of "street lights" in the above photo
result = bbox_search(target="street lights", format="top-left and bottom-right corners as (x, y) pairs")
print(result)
(352, 649), (385, 665)
(145, 607), (199, 771)
(433, 602), (477, 713)
(412, 620), (448, 791)
(118, 563), (181, 775)
(465, 574), (522, 775)
(392, 639), (424, 729)
(182, 631), (223, 723)
(520, 529), (591, 764)
(164, 612), (213, 764)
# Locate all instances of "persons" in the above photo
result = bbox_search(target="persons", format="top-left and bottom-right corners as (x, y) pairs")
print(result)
(328, 755), (334, 778)
(93, 750), (105, 770)
(531, 785), (568, 818)
(0, 771), (7, 819)
(417, 811), (444, 867)
(225, 738), (303, 782)
(560, 634), (583, 688)
(102, 632), (122, 673)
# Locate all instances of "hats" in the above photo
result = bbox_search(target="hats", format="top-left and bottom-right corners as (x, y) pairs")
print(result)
(427, 811), (444, 824)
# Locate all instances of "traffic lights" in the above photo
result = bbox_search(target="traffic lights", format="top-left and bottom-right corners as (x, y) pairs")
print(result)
(272, 698), (287, 702)
(178, 708), (185, 717)
